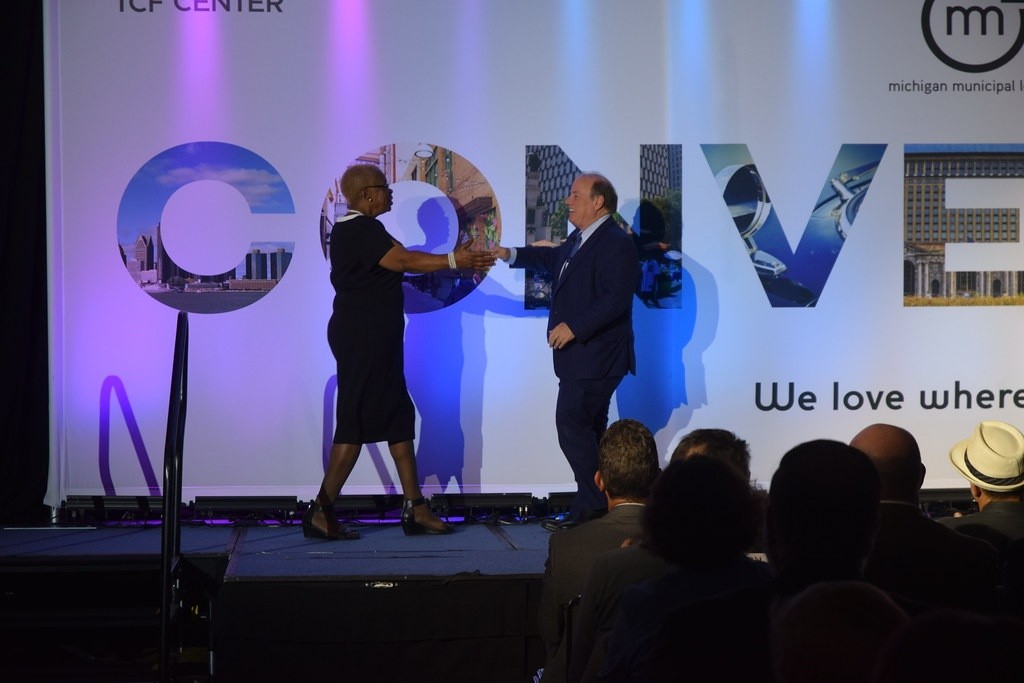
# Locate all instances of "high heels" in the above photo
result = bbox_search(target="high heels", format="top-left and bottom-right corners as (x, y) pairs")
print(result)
(301, 499), (361, 540)
(402, 497), (463, 536)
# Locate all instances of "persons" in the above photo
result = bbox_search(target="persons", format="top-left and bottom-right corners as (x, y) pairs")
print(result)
(301, 164), (496, 540)
(484, 172), (640, 533)
(535, 419), (1024, 683)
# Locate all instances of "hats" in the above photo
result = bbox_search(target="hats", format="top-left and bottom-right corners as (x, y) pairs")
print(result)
(949, 421), (1024, 492)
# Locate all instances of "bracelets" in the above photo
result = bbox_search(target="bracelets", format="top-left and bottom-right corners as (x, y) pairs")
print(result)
(447, 252), (456, 269)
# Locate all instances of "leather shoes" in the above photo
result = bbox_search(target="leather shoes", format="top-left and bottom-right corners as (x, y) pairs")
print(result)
(542, 513), (606, 532)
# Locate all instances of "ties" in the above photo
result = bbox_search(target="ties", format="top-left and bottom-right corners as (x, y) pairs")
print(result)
(567, 233), (582, 262)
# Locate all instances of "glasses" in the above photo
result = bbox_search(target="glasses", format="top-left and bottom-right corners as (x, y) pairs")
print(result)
(362, 181), (389, 191)
